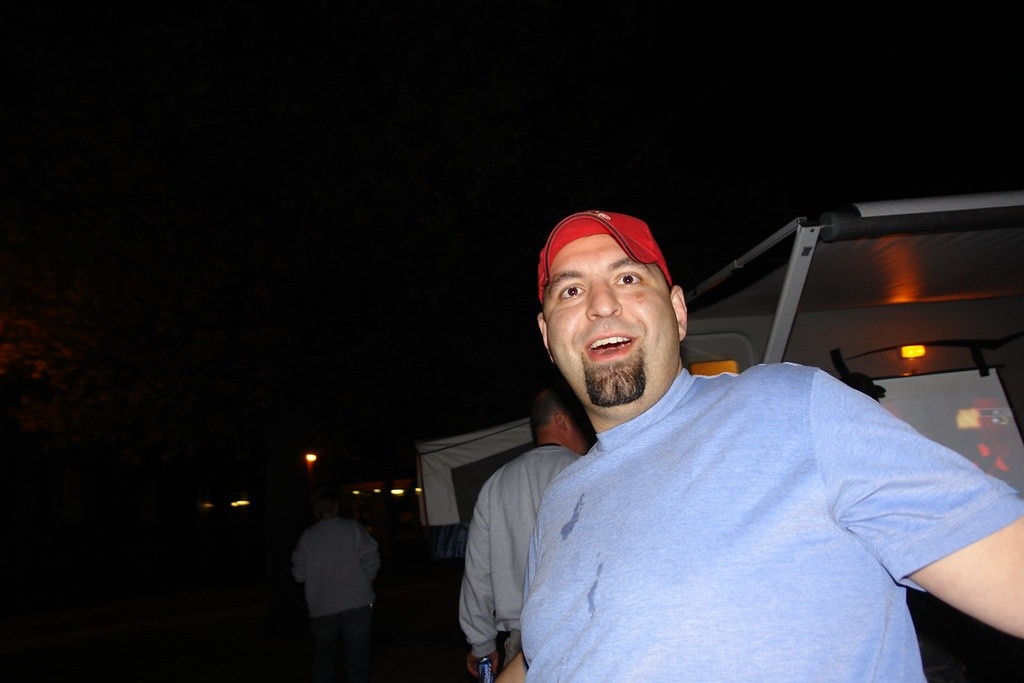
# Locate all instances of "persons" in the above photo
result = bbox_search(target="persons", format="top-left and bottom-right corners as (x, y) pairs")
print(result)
(291, 487), (381, 683)
(459, 388), (592, 683)
(494, 209), (1024, 683)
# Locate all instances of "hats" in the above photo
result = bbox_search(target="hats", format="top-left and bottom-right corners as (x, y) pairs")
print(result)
(537, 210), (672, 303)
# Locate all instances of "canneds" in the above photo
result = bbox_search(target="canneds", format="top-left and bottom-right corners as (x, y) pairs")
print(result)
(477, 656), (494, 683)
